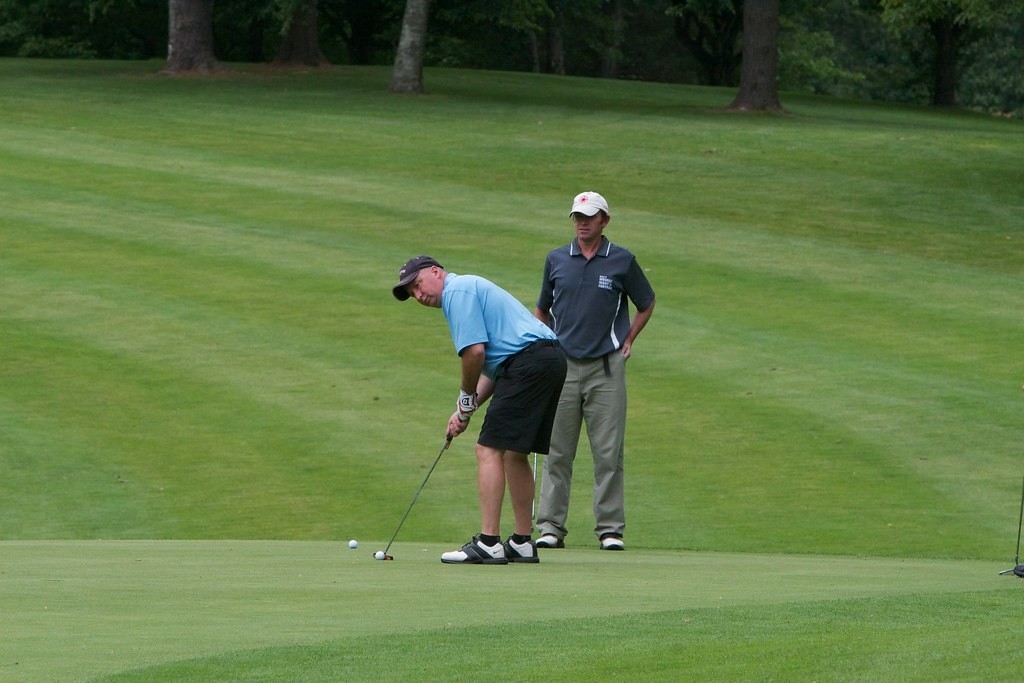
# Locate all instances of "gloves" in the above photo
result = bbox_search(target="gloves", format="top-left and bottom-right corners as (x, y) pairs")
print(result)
(455, 386), (479, 422)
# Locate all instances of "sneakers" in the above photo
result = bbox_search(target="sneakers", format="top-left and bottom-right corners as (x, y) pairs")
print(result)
(503, 535), (539, 562)
(599, 534), (625, 550)
(534, 534), (564, 548)
(440, 533), (509, 565)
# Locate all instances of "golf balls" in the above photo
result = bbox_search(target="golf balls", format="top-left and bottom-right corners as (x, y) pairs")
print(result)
(375, 550), (385, 560)
(348, 539), (358, 548)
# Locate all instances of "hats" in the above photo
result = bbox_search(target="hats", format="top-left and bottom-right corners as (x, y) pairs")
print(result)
(392, 255), (444, 301)
(568, 190), (609, 217)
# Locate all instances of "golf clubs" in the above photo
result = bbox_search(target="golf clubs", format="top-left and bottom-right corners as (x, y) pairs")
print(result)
(997, 486), (1024, 576)
(372, 418), (463, 560)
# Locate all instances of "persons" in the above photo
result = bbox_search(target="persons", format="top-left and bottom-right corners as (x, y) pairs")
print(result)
(386, 255), (568, 564)
(535, 192), (656, 550)
